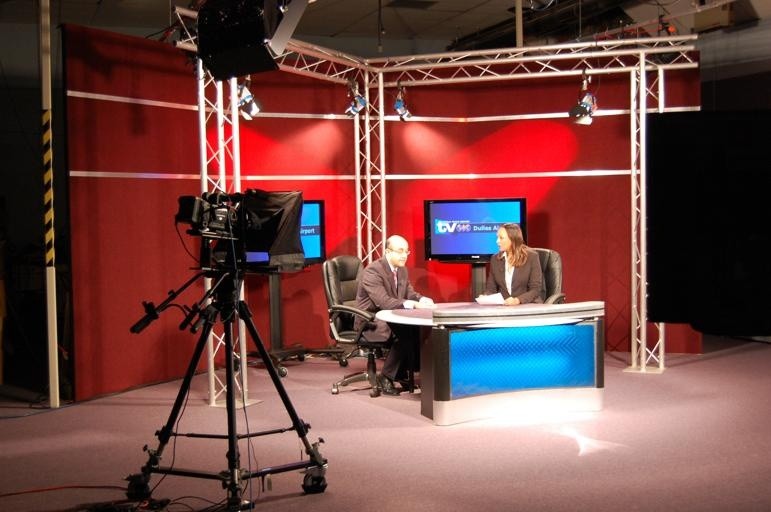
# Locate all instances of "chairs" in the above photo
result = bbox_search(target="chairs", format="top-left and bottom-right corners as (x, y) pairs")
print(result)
(531, 247), (566, 304)
(322, 255), (399, 398)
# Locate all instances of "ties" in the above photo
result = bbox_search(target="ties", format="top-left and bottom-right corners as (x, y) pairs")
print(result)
(393, 270), (398, 289)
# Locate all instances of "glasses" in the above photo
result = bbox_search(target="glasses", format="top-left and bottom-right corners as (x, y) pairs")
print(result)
(388, 246), (412, 256)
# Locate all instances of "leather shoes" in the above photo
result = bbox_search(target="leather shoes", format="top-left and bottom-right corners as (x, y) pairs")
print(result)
(376, 371), (419, 396)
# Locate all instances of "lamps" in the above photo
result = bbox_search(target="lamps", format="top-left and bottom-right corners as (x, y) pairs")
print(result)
(237, 80), (260, 121)
(345, 83), (366, 119)
(568, 76), (598, 124)
(393, 88), (412, 123)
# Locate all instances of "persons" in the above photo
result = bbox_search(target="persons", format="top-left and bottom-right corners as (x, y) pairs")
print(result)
(355, 235), (435, 398)
(481, 223), (548, 303)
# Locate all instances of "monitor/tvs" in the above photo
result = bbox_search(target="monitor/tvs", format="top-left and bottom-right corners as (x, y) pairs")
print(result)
(245, 199), (326, 269)
(423, 198), (526, 262)
